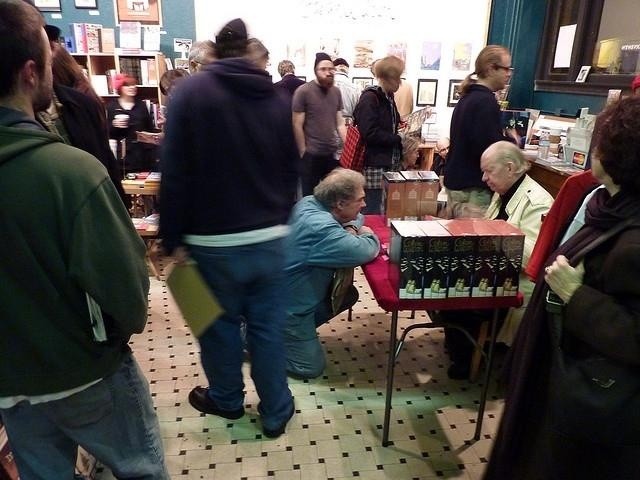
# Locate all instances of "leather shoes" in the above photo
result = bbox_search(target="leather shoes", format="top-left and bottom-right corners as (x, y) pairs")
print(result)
(186, 386), (247, 421)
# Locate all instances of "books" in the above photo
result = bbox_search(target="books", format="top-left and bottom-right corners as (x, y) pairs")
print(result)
(118, 22), (160, 51)
(121, 170), (163, 191)
(139, 59), (156, 86)
(69, 22), (116, 54)
(165, 256), (228, 341)
(83, 292), (123, 343)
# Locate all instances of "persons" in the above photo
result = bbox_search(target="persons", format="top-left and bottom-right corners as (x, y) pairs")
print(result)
(0, 0), (172, 480)
(270, 58), (304, 107)
(445, 43), (521, 224)
(351, 56), (407, 216)
(104, 75), (152, 220)
(157, 67), (192, 127)
(51, 40), (132, 220)
(445, 144), (556, 382)
(295, 50), (346, 197)
(238, 166), (379, 380)
(370, 60), (412, 122)
(487, 96), (640, 480)
(331, 56), (358, 132)
(380, 134), (450, 219)
(104, 71), (152, 220)
(154, 20), (299, 437)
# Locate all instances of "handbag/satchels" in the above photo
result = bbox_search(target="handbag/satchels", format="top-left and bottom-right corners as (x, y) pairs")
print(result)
(533, 218), (640, 480)
(336, 122), (364, 170)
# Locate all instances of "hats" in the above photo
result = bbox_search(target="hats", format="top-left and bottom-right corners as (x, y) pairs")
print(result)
(314, 53), (332, 68)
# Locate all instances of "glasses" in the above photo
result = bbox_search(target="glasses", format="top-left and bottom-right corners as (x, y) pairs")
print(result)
(492, 62), (515, 75)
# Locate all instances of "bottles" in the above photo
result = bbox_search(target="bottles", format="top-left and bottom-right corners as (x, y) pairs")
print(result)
(538, 131), (551, 160)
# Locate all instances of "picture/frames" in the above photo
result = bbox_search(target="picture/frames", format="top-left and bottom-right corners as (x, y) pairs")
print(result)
(74, 0), (98, 9)
(32, 0), (62, 12)
(570, 151), (588, 169)
(295, 76), (306, 82)
(575, 65), (592, 82)
(174, 58), (189, 73)
(113, 0), (163, 28)
(447, 79), (465, 107)
(173, 38), (192, 52)
(416, 79), (438, 107)
(352, 77), (373, 98)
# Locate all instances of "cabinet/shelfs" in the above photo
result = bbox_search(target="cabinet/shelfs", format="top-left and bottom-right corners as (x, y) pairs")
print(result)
(69, 52), (166, 179)
(416, 144), (436, 171)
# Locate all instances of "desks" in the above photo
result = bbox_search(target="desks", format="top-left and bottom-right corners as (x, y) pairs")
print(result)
(348, 215), (524, 448)
(140, 230), (161, 281)
(121, 178), (161, 218)
(520, 150), (589, 200)
